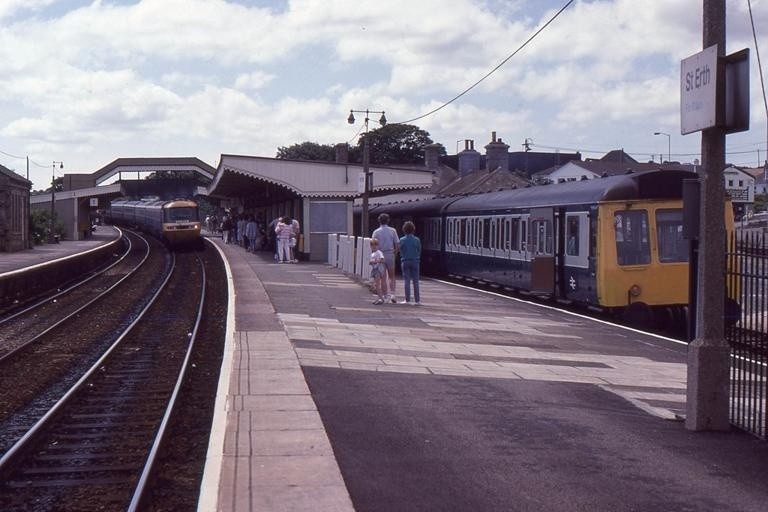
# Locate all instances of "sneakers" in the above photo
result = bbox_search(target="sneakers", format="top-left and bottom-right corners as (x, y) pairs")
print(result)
(372, 295), (424, 306)
(278, 259), (299, 264)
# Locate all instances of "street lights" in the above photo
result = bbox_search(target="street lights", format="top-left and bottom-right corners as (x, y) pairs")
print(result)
(654, 132), (671, 161)
(347, 109), (386, 236)
(50, 162), (64, 243)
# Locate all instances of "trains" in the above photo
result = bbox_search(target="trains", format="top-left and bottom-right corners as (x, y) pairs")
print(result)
(355, 166), (742, 333)
(105, 195), (201, 251)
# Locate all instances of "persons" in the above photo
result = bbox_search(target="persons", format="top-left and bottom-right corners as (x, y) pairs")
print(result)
(205, 211), (300, 265)
(399, 221), (422, 305)
(368, 237), (386, 305)
(372, 213), (400, 304)
